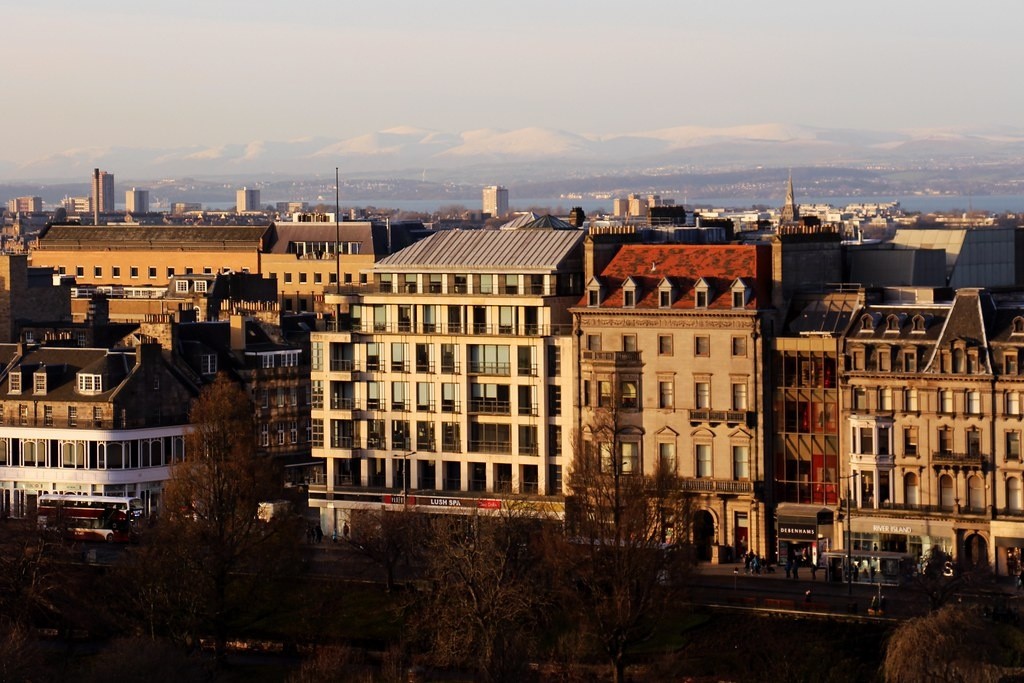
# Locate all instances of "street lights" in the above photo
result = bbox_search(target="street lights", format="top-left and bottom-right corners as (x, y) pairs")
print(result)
(842, 471), (861, 607)
(393, 451), (417, 585)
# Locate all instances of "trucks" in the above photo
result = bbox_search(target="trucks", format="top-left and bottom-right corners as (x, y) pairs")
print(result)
(257, 499), (304, 539)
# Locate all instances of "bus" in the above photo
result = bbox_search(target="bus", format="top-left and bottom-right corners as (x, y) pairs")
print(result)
(36, 505), (134, 546)
(37, 494), (142, 525)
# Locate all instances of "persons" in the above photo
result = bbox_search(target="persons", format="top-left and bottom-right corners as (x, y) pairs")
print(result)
(785, 560), (792, 578)
(743, 550), (766, 576)
(792, 560), (800, 579)
(306, 525), (324, 546)
(874, 543), (878, 551)
(342, 522), (349, 542)
(853, 565), (859, 582)
(881, 567), (888, 583)
(332, 528), (338, 544)
(870, 565), (876, 582)
(811, 563), (818, 580)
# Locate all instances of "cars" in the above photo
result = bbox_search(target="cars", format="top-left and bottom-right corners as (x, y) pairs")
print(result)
(867, 595), (886, 618)
